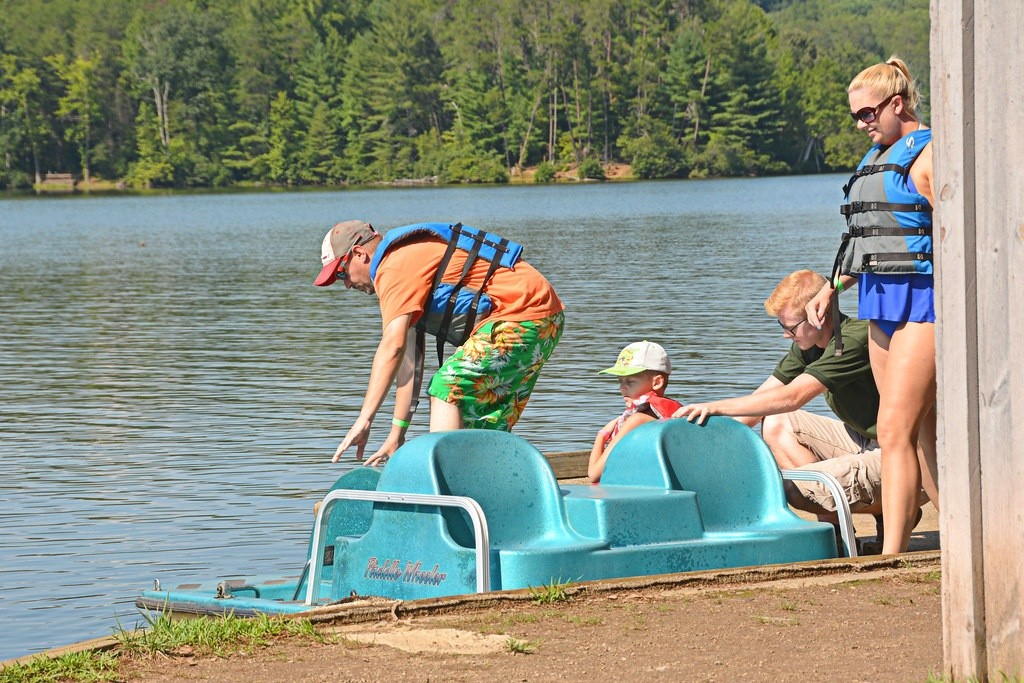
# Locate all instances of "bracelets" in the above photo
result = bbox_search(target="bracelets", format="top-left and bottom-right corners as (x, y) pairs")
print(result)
(830, 277), (845, 294)
(596, 430), (610, 440)
(391, 417), (410, 429)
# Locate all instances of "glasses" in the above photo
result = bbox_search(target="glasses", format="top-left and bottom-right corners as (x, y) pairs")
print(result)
(777, 319), (807, 337)
(336, 236), (361, 279)
(848, 93), (907, 123)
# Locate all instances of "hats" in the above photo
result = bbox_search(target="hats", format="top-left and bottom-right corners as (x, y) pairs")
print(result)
(598, 340), (671, 376)
(313, 220), (380, 286)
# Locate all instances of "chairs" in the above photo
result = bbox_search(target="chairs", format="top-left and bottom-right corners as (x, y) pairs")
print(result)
(297, 407), (839, 599)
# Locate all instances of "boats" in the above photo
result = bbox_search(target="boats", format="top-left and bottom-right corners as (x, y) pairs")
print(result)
(132, 412), (860, 618)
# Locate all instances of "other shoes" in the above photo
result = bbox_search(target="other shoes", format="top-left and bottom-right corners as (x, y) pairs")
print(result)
(863, 506), (922, 556)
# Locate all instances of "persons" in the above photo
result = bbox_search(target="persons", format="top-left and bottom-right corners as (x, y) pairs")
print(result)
(587, 340), (684, 482)
(313, 220), (565, 465)
(671, 269), (932, 559)
(805, 54), (942, 556)
